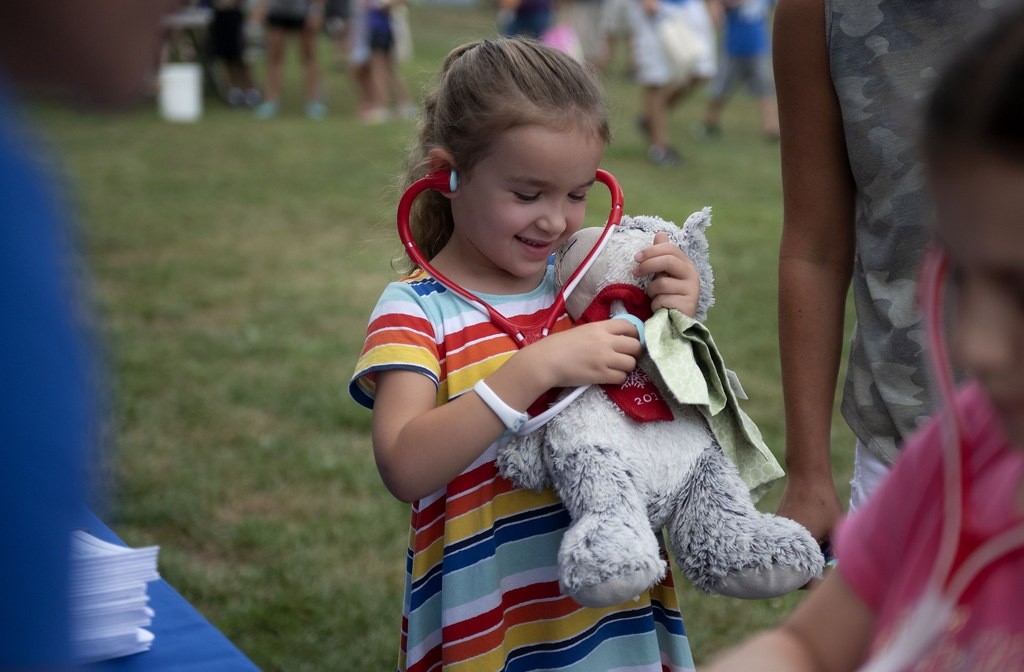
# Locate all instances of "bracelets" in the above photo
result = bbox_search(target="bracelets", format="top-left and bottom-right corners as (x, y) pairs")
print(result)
(476, 379), (528, 434)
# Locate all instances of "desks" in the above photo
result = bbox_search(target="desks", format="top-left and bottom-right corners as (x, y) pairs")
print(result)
(67, 500), (260, 672)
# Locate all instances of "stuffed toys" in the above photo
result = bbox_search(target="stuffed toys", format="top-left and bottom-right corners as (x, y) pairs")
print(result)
(494, 207), (825, 608)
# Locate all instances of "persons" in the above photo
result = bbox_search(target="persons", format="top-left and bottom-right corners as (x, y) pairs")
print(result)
(244, 0), (328, 121)
(347, 38), (701, 672)
(614, 1), (720, 164)
(702, 1), (784, 142)
(2, 106), (98, 670)
(198, 0), (256, 108)
(772, 2), (1024, 592)
(494, 0), (555, 40)
(345, 0), (421, 124)
(700, 23), (1023, 672)
